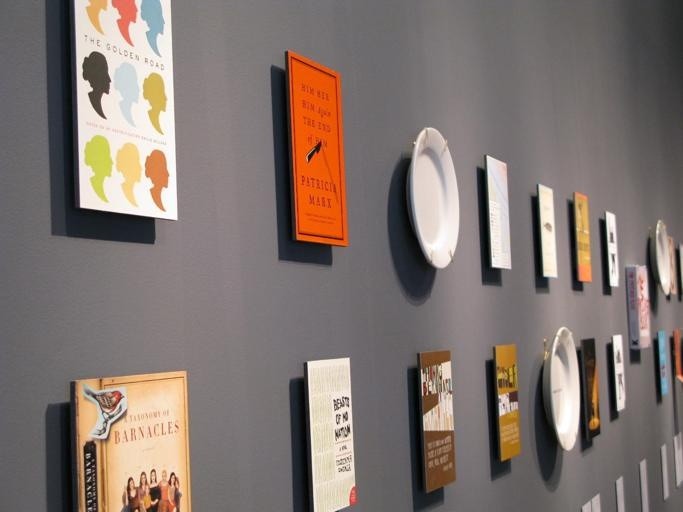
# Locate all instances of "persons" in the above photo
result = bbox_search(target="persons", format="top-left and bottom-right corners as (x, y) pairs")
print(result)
(121, 467), (179, 512)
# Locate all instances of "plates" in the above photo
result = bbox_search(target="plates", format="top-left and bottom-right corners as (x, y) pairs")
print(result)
(541, 326), (582, 452)
(404, 126), (461, 269)
(648, 219), (672, 297)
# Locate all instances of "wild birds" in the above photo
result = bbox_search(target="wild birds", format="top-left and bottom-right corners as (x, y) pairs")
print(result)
(83, 387), (125, 420)
